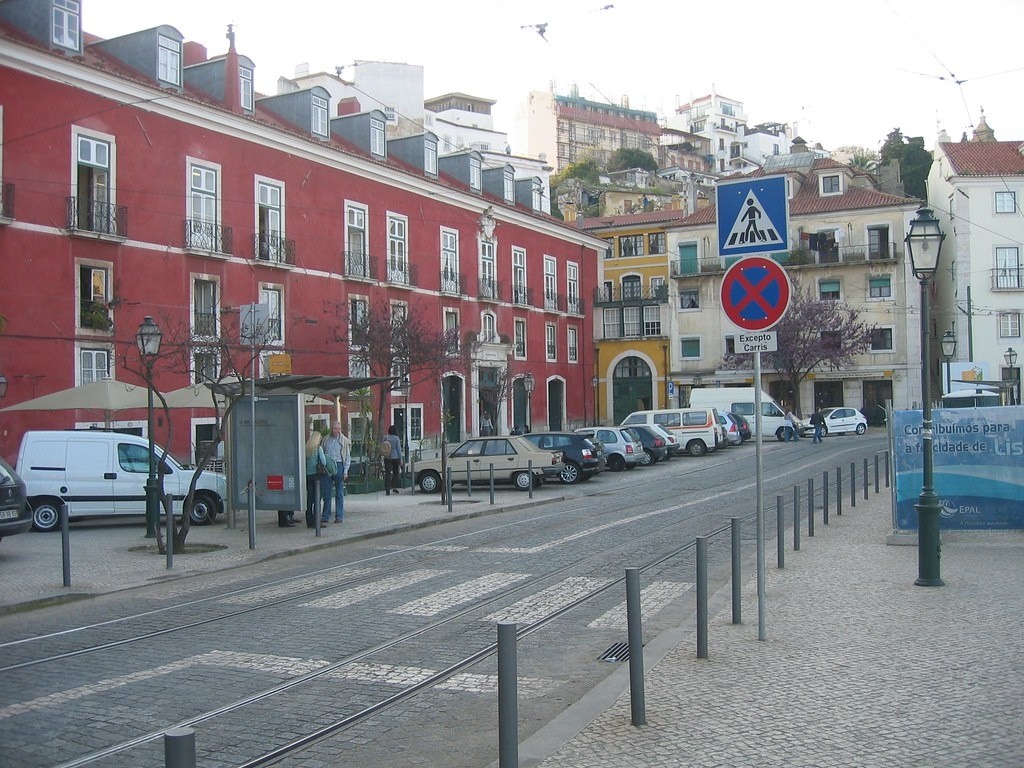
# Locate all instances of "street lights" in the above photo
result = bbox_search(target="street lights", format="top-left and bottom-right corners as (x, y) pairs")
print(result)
(522, 372), (535, 431)
(134, 314), (167, 538)
(902, 207), (950, 588)
(940, 329), (958, 393)
(1003, 347), (1018, 407)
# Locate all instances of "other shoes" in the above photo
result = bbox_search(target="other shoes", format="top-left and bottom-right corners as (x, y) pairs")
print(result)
(334, 519), (342, 523)
(386, 490), (391, 495)
(820, 440), (823, 443)
(307, 522), (314, 528)
(393, 489), (399, 493)
(313, 523), (327, 528)
(278, 518), (302, 527)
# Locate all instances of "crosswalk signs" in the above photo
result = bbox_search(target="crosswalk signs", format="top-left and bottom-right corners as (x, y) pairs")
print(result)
(714, 173), (789, 257)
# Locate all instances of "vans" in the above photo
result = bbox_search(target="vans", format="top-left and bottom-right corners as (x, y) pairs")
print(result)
(614, 407), (729, 456)
(689, 387), (805, 441)
(13, 430), (227, 534)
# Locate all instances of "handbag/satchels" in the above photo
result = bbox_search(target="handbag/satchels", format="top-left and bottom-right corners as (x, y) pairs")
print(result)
(316, 447), (327, 475)
(380, 435), (391, 457)
(792, 423), (796, 428)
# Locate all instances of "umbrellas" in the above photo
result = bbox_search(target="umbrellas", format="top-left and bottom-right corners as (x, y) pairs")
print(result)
(121, 373), (347, 408)
(0, 376), (166, 429)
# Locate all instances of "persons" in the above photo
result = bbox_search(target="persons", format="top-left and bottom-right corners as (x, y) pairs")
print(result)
(811, 406), (827, 444)
(320, 421), (353, 524)
(523, 425), (530, 434)
(277, 510), (302, 528)
(304, 431), (328, 529)
(510, 426), (523, 435)
(783, 406), (799, 443)
(380, 425), (403, 496)
(480, 411), (493, 436)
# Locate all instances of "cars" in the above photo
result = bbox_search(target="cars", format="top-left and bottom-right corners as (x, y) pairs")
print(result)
(801, 406), (869, 437)
(520, 431), (607, 486)
(571, 426), (645, 472)
(621, 423), (679, 468)
(730, 412), (753, 443)
(665, 412), (741, 451)
(405, 436), (567, 494)
(0, 457), (35, 542)
